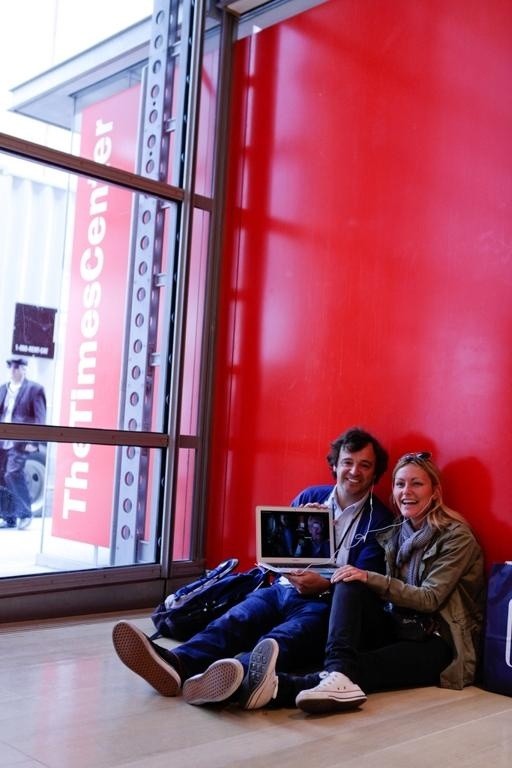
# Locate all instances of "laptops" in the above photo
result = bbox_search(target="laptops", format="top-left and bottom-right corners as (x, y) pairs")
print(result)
(256, 505), (342, 574)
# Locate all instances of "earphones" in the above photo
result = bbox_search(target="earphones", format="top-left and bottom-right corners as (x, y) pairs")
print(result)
(432, 491), (436, 497)
(373, 475), (376, 481)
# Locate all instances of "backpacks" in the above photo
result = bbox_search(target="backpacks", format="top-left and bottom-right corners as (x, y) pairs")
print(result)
(149, 558), (267, 638)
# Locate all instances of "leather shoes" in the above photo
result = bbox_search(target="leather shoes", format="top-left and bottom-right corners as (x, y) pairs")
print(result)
(19, 517), (30, 530)
(0, 519), (17, 527)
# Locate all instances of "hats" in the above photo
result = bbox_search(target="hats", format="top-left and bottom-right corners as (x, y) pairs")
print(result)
(7, 357), (28, 365)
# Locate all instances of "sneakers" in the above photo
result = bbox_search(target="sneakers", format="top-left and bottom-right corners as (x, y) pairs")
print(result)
(110, 621), (368, 716)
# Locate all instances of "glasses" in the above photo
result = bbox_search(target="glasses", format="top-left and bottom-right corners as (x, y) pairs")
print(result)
(400, 451), (432, 463)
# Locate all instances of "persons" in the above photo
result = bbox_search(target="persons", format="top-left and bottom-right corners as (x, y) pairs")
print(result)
(236, 450), (487, 716)
(295, 515), (329, 558)
(1, 356), (48, 531)
(111, 426), (395, 705)
